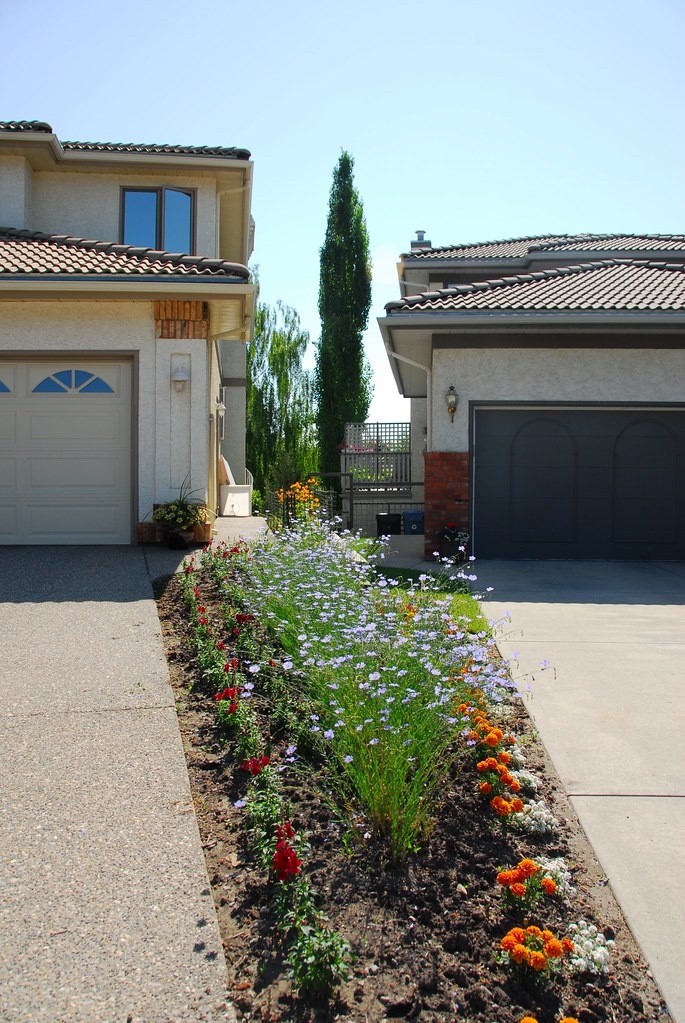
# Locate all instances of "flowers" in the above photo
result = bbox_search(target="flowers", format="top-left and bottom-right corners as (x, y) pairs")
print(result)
(430, 521), (466, 541)
(141, 470), (219, 547)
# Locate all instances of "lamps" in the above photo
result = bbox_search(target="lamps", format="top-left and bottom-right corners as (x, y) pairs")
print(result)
(217, 402), (226, 418)
(170, 366), (191, 397)
(445, 383), (459, 423)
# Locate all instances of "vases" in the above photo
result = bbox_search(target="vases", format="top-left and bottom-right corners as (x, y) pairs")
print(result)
(164, 531), (194, 550)
(442, 540), (463, 564)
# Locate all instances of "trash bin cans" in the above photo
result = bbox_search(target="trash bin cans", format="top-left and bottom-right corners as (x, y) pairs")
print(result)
(402, 511), (425, 535)
(375, 513), (401, 537)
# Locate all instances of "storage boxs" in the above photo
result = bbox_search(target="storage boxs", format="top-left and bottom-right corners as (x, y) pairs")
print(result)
(219, 484), (252, 516)
